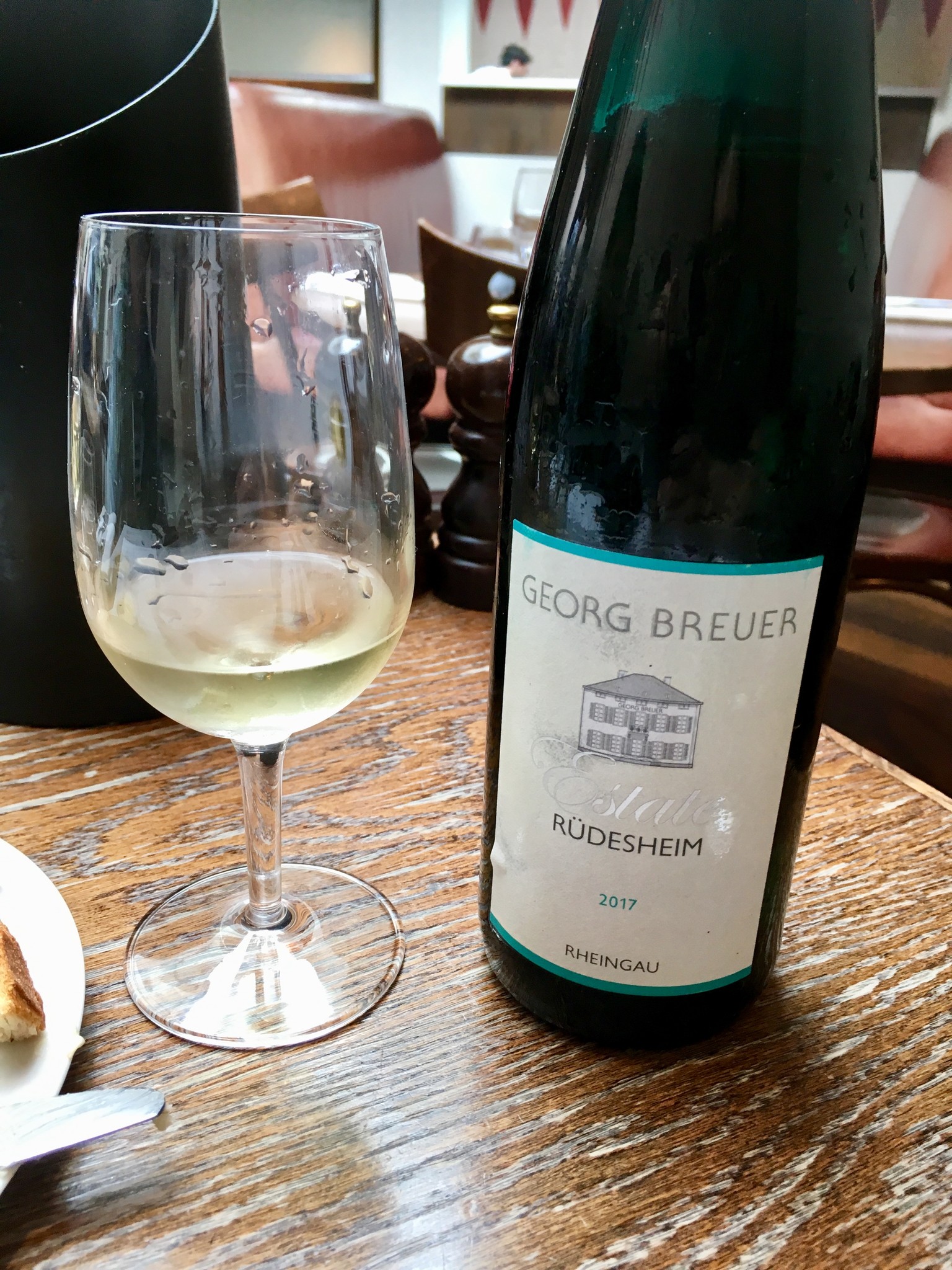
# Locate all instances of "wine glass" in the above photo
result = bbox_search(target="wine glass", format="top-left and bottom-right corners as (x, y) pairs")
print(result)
(68, 211), (414, 1051)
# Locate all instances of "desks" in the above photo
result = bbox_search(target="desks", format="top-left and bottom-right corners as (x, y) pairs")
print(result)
(0, 583), (952, 1270)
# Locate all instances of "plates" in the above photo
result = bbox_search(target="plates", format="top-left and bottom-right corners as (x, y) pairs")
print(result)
(0, 839), (86, 1196)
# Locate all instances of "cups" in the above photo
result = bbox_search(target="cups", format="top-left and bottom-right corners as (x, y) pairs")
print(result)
(514, 172), (554, 266)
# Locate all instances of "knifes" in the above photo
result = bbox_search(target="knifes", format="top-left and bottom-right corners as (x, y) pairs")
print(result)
(0, 1086), (165, 1168)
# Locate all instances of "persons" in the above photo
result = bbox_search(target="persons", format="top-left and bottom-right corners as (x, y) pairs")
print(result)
(465, 44), (531, 88)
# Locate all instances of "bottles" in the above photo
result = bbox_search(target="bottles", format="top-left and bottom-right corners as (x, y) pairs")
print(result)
(1, 1), (243, 729)
(479, 0), (887, 1056)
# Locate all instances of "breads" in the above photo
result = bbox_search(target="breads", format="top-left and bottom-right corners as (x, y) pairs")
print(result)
(0, 922), (46, 1042)
